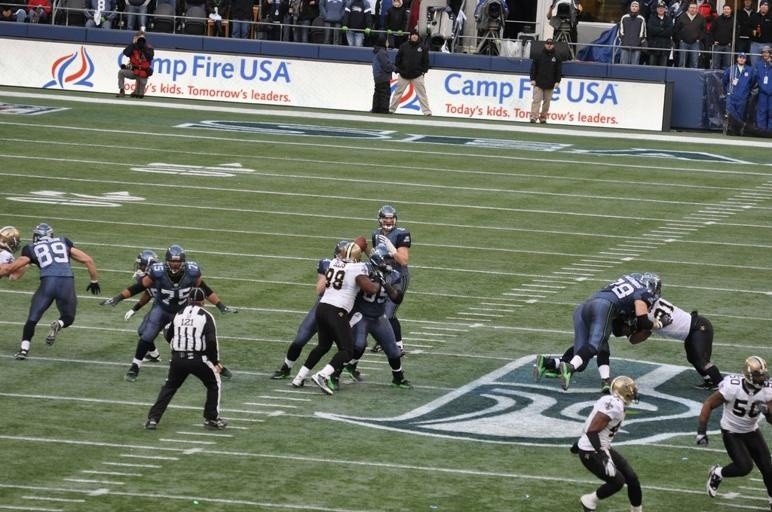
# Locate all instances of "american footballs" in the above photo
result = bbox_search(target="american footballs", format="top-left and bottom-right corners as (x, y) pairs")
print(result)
(353, 234), (367, 252)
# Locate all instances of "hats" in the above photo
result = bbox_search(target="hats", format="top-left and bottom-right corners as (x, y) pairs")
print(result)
(189, 287), (205, 301)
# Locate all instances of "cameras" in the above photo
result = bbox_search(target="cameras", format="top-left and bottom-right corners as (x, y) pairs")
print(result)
(134, 37), (145, 49)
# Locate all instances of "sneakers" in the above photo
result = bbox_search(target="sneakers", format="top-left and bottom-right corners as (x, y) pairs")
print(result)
(580, 494), (596, 512)
(274, 342), (413, 394)
(533, 355), (611, 392)
(222, 306), (237, 315)
(46, 321), (60, 345)
(13, 349), (28, 360)
(125, 353), (233, 430)
(694, 378), (723, 390)
(707, 464), (722, 498)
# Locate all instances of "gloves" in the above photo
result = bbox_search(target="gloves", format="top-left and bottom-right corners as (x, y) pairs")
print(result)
(659, 315), (674, 328)
(87, 280), (135, 322)
(696, 434), (708, 445)
(599, 448), (617, 477)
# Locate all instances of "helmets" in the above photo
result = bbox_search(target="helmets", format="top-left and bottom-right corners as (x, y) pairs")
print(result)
(135, 250), (161, 273)
(32, 222), (54, 242)
(377, 206), (397, 231)
(742, 356), (769, 389)
(165, 245), (186, 269)
(335, 241), (361, 264)
(611, 375), (639, 407)
(0, 225), (21, 253)
(633, 272), (661, 295)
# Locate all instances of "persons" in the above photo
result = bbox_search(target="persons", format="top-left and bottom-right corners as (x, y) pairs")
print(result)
(574, 375), (651, 512)
(694, 355), (772, 503)
(1, 224), (103, 359)
(720, 45), (771, 134)
(372, 37), (400, 113)
(116, 32), (154, 98)
(620, 0), (772, 69)
(530, 268), (662, 390)
(371, 206), (410, 353)
(101, 245), (239, 381)
(0, 0), (407, 48)
(530, 0), (582, 124)
(390, 32), (432, 116)
(1, 225), (30, 281)
(609, 297), (725, 392)
(143, 288), (227, 429)
(271, 238), (410, 395)
